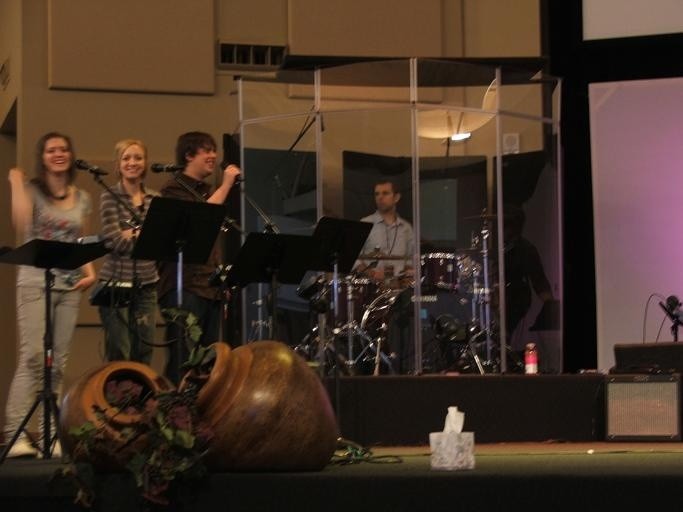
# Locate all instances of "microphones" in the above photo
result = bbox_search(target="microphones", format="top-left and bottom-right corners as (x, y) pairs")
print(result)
(220, 158), (243, 185)
(76, 158), (106, 176)
(148, 162), (186, 174)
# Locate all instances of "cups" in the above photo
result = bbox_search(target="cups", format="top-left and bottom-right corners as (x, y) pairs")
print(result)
(427, 429), (477, 472)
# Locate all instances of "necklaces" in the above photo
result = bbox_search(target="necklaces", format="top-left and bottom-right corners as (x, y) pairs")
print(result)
(47, 184), (72, 199)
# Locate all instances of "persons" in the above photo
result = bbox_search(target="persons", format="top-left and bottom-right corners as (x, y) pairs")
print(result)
(96, 138), (163, 365)
(350, 176), (412, 374)
(1, 133), (97, 463)
(495, 199), (556, 342)
(154, 132), (241, 389)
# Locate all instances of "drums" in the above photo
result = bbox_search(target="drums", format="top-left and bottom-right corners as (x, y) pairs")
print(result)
(360, 288), (470, 371)
(397, 270), (417, 286)
(327, 277), (390, 337)
(418, 252), (465, 291)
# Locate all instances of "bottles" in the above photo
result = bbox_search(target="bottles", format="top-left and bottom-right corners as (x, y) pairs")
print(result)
(524, 342), (539, 374)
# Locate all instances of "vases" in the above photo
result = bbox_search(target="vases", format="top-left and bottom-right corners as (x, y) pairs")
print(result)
(177, 336), (340, 469)
(50, 360), (176, 484)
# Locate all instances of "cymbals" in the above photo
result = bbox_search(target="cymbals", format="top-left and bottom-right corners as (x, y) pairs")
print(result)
(355, 252), (408, 260)
(460, 213), (508, 222)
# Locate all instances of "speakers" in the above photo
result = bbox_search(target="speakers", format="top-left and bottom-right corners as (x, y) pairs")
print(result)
(603, 373), (681, 442)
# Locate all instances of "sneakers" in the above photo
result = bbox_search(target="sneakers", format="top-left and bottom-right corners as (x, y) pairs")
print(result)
(5, 428), (63, 458)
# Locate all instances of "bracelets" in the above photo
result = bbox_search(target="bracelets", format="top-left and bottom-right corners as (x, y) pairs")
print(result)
(131, 225), (140, 241)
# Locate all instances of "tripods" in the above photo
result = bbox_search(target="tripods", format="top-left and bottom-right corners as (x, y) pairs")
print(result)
(319, 279), (389, 374)
(1, 275), (62, 463)
(465, 246), (521, 373)
